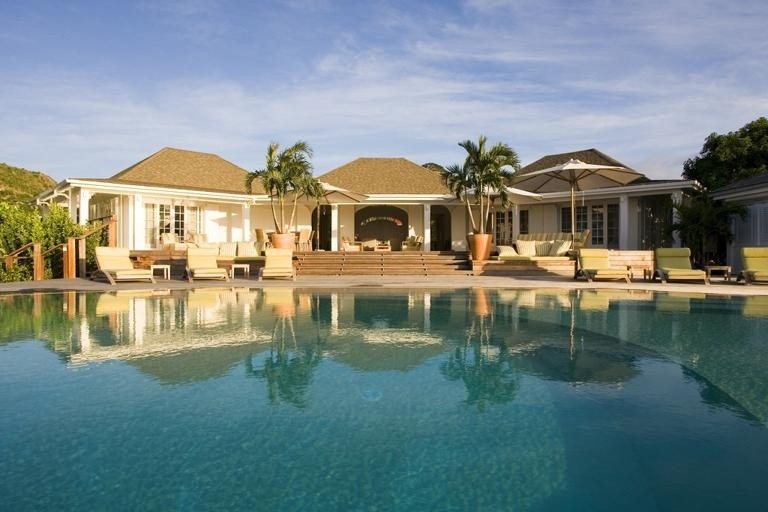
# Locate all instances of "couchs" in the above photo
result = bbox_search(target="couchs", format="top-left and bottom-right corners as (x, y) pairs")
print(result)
(512, 228), (592, 255)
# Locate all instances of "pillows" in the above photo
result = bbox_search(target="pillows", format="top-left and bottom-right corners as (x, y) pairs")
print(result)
(494, 239), (571, 257)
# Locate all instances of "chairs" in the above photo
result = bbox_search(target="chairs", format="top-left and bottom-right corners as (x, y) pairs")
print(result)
(340, 233), (424, 253)
(574, 246), (768, 286)
(89, 226), (317, 286)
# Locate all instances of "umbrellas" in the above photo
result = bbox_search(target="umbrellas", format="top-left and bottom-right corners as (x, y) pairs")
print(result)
(501, 147), (647, 229)
(436, 182), (545, 210)
(510, 312), (645, 389)
(267, 178), (371, 226)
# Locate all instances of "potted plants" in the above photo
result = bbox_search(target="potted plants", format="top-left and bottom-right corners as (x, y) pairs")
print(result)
(439, 133), (522, 262)
(245, 299), (329, 410)
(441, 287), (521, 416)
(242, 138), (330, 250)
